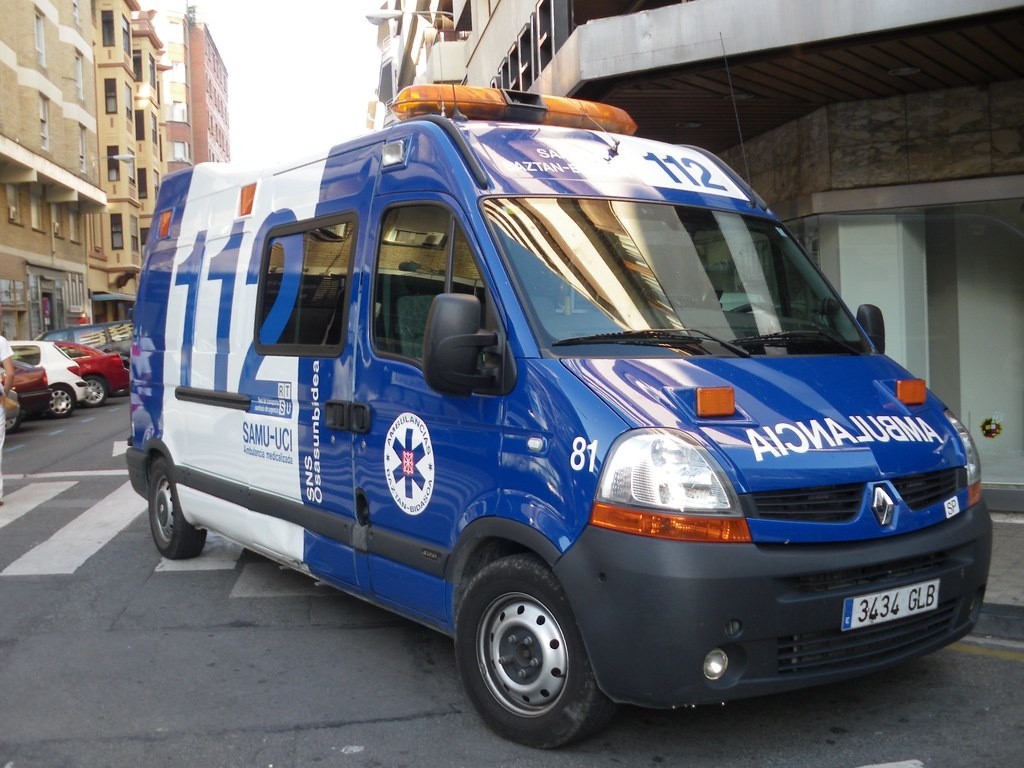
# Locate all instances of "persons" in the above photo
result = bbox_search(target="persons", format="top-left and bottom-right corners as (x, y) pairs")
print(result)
(0, 335), (15, 505)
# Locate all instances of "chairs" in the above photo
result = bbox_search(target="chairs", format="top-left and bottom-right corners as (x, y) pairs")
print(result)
(397, 294), (441, 362)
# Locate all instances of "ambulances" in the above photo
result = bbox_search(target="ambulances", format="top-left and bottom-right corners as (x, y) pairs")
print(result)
(126, 84), (993, 750)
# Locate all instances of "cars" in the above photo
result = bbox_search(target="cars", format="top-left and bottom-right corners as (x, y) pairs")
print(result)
(0, 359), (53, 434)
(8, 340), (88, 419)
(52, 339), (130, 407)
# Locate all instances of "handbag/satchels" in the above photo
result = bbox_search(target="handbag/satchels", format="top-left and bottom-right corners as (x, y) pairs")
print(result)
(2, 389), (19, 418)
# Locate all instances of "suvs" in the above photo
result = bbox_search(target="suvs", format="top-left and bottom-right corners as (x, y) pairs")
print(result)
(33, 320), (134, 397)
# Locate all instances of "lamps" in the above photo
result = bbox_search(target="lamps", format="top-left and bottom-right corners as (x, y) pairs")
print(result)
(86, 154), (136, 165)
(364, 10), (455, 27)
